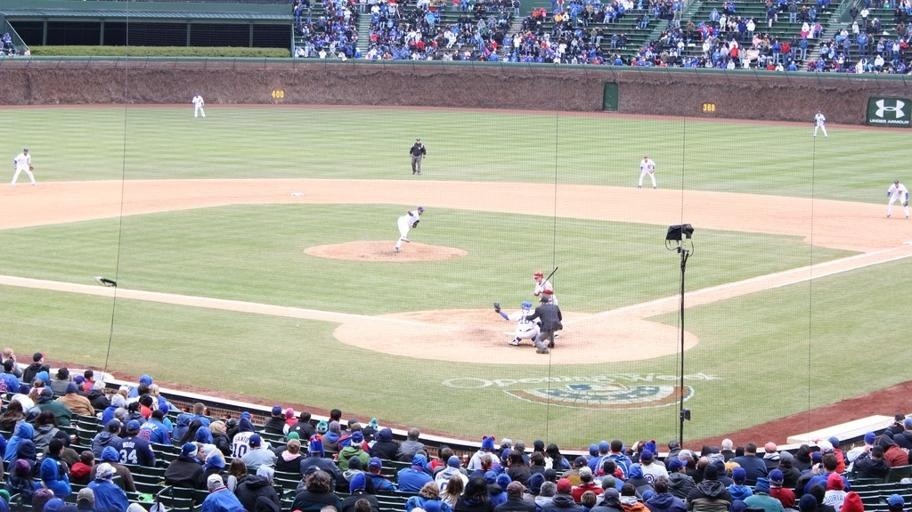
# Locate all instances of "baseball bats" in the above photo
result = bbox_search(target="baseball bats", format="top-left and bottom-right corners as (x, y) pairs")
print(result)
(540, 267), (559, 286)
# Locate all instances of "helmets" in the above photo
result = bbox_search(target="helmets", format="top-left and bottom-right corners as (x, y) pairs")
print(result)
(521, 302), (532, 309)
(534, 272), (543, 278)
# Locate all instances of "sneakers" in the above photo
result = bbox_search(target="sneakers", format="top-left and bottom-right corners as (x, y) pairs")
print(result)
(508, 339), (519, 346)
(537, 349), (548, 353)
(548, 342), (554, 348)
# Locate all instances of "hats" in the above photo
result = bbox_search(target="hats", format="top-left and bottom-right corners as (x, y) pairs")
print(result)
(412, 455), (427, 468)
(105, 375), (170, 432)
(799, 438), (863, 511)
(182, 411), (273, 489)
(31, 431), (118, 508)
(447, 436), (618, 501)
(539, 297), (548, 303)
(865, 414), (912, 507)
(31, 354), (106, 400)
(272, 407), (392, 493)
(640, 438), (793, 493)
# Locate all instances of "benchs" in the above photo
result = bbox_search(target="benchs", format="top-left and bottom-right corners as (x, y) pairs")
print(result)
(369, 4), (515, 54)
(521, 0), (912, 72)
(293, 3), (326, 50)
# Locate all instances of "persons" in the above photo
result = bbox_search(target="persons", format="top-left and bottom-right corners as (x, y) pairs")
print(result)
(812, 109), (828, 137)
(409, 136), (427, 176)
(886, 179), (909, 220)
(532, 271), (560, 339)
(1, 348), (912, 511)
(494, 300), (541, 348)
(10, 147), (36, 185)
(0, 31), (30, 56)
(393, 206), (427, 253)
(192, 92), (206, 119)
(525, 295), (563, 355)
(637, 156), (657, 190)
(293, 0), (912, 77)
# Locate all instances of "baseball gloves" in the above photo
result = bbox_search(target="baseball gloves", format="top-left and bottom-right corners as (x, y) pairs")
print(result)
(494, 304), (500, 313)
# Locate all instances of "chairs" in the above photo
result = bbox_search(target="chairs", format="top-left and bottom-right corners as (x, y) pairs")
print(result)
(789, 464), (912, 512)
(0, 393), (420, 512)
